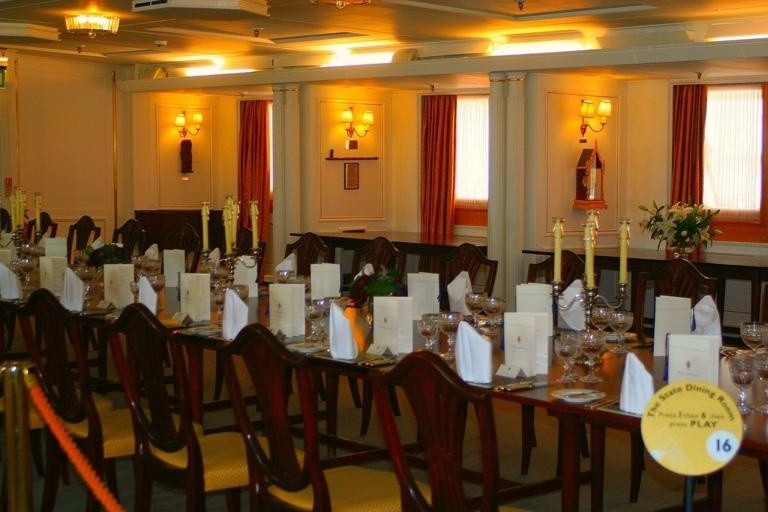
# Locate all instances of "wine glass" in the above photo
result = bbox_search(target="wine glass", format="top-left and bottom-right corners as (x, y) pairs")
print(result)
(551, 303), (633, 384)
(275, 267), (348, 340)
(419, 291), (505, 365)
(128, 252), (248, 318)
(12, 239), (96, 314)
(726, 318), (768, 417)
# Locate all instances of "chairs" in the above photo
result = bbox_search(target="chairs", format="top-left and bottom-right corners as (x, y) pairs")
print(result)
(494, 227), (495, 239)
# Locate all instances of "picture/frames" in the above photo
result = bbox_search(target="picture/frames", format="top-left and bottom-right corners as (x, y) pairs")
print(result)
(344, 162), (359, 190)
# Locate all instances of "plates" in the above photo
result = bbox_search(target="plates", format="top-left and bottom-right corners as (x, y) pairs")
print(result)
(597, 332), (637, 341)
(187, 325), (224, 336)
(287, 341), (324, 354)
(550, 389), (607, 404)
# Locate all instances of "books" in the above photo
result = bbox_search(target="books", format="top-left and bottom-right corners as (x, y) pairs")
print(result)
(407, 271), (438, 320)
(654, 295), (689, 357)
(666, 334), (719, 392)
(372, 296), (413, 355)
(514, 283), (555, 336)
(504, 311), (548, 379)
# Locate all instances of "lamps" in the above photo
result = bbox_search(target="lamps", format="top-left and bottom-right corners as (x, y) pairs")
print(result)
(56, 3), (123, 46)
(175, 111), (203, 137)
(342, 106), (375, 138)
(576, 98), (612, 143)
